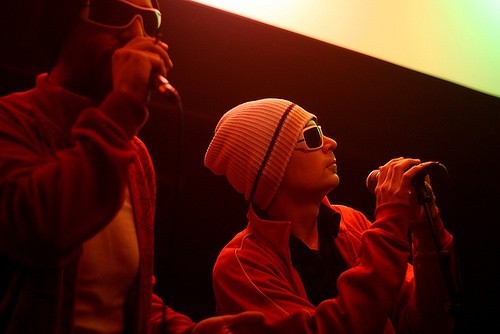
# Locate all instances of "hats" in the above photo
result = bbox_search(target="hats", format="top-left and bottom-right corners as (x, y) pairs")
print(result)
(204, 98), (318, 211)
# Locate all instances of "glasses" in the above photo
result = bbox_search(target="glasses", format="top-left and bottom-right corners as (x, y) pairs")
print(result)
(81, 0), (161, 41)
(297, 125), (323, 150)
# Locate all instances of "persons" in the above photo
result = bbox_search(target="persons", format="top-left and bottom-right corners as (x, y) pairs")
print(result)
(205, 98), (458, 334)
(1, 0), (263, 333)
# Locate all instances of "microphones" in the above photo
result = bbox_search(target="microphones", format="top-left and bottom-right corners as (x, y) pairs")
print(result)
(149, 71), (179, 104)
(365, 161), (441, 194)
